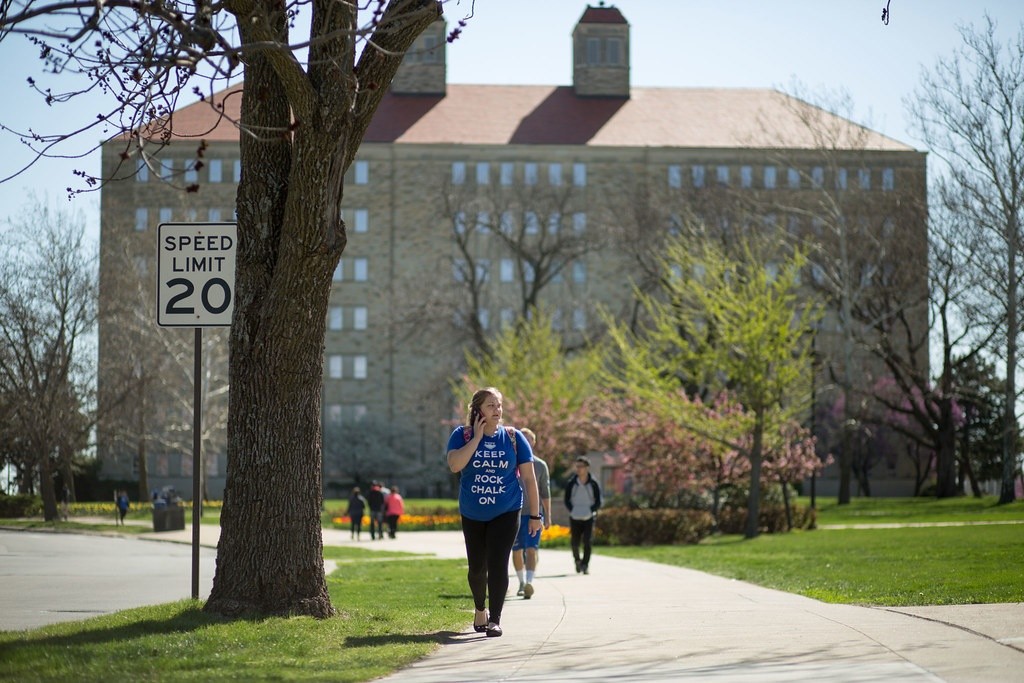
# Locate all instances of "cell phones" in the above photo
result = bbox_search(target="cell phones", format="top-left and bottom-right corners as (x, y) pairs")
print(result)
(472, 406), (486, 423)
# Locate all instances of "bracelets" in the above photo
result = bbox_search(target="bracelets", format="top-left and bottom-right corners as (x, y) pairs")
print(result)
(530, 515), (541, 519)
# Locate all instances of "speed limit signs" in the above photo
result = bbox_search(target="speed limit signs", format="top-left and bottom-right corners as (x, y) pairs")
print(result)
(155, 221), (236, 326)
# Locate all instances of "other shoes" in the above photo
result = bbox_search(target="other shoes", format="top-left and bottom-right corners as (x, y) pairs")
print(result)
(576, 559), (581, 573)
(581, 560), (588, 574)
(517, 585), (525, 595)
(486, 626), (502, 636)
(524, 583), (534, 599)
(474, 613), (489, 632)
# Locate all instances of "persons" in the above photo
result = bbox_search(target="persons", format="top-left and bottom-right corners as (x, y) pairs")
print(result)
(61, 498), (70, 521)
(368, 481), (404, 539)
(512, 428), (551, 599)
(564, 456), (601, 575)
(446, 389), (542, 637)
(117, 491), (130, 525)
(344, 489), (366, 540)
(153, 489), (181, 508)
